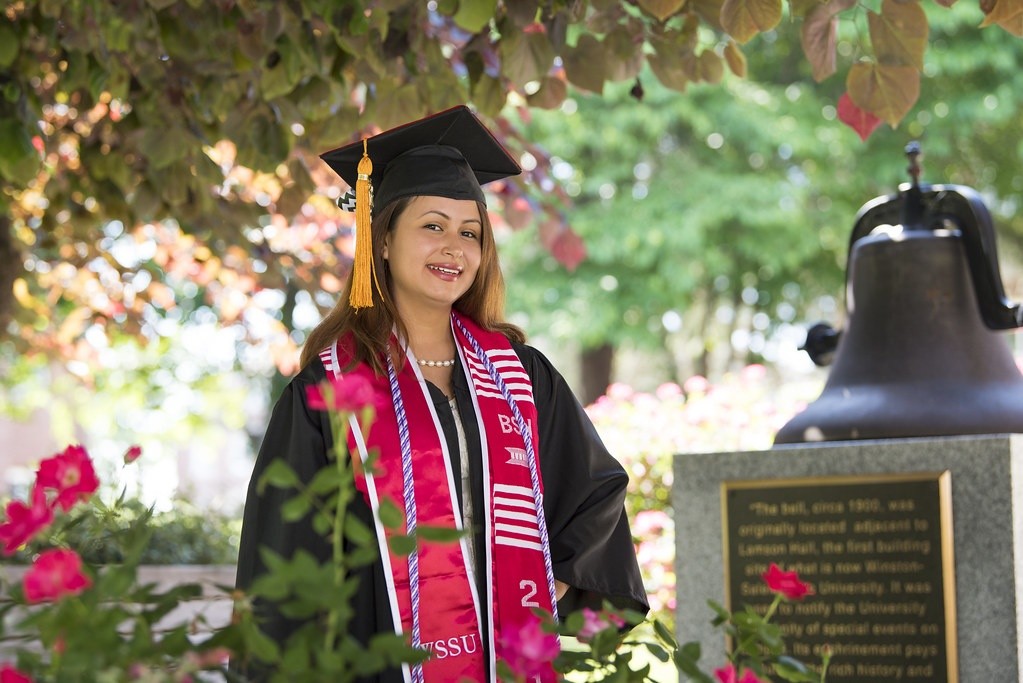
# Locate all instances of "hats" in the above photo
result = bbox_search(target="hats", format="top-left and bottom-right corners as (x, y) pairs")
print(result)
(319, 105), (523, 315)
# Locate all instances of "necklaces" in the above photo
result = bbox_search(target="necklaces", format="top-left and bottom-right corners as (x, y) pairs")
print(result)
(414, 358), (455, 368)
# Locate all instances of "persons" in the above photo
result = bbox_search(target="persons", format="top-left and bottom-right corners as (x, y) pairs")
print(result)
(226, 102), (650, 683)
